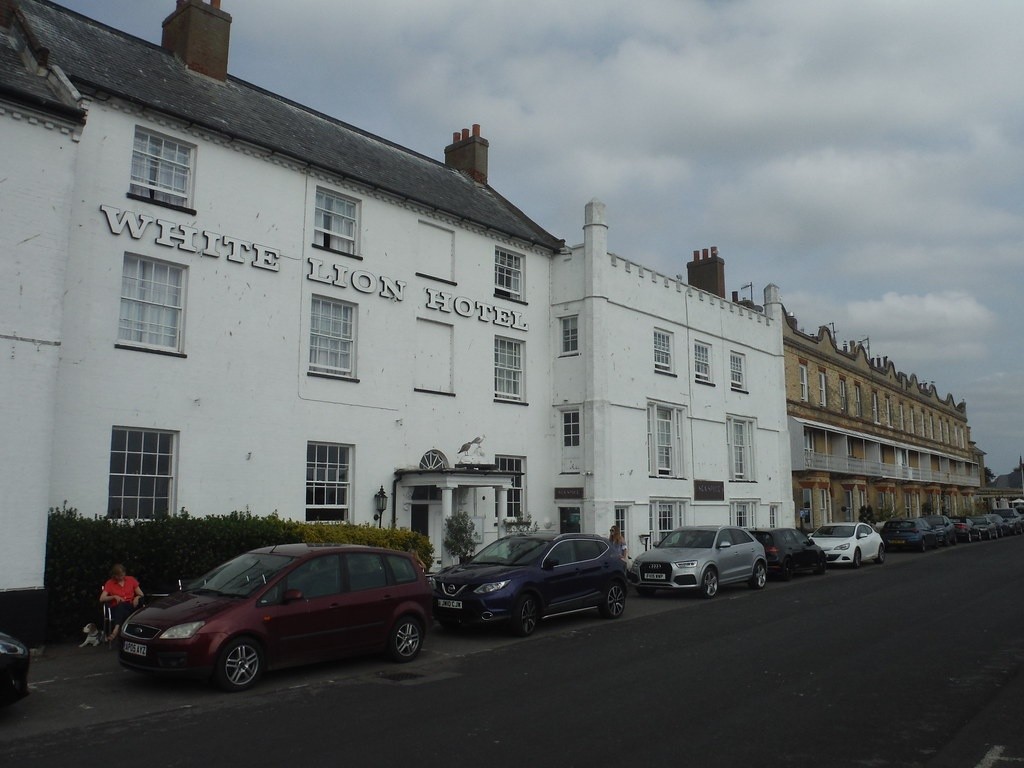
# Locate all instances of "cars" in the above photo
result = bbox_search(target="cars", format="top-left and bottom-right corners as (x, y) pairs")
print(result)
(807, 522), (886, 568)
(629, 525), (769, 599)
(0, 629), (30, 706)
(919, 514), (957, 547)
(949, 506), (1024, 543)
(747, 526), (827, 581)
(879, 517), (939, 553)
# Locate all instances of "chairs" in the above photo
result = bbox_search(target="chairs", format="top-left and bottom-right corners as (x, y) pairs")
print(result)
(289, 561), (336, 596)
(102, 587), (147, 650)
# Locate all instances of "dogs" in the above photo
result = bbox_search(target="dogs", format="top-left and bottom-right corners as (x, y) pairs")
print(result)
(78, 623), (120, 648)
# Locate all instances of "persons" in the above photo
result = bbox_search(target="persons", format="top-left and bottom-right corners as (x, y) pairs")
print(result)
(609, 526), (629, 562)
(409, 549), (427, 570)
(100, 564), (144, 642)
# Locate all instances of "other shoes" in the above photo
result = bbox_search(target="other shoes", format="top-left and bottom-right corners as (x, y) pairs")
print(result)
(107, 635), (116, 642)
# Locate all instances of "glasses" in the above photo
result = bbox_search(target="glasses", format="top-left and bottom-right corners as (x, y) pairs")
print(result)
(610, 529), (614, 532)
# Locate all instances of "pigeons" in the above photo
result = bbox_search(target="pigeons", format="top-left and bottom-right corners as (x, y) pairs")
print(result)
(457, 442), (472, 456)
(468, 434), (486, 448)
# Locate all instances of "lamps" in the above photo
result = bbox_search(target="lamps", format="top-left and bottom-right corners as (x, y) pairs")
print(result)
(374, 486), (388, 528)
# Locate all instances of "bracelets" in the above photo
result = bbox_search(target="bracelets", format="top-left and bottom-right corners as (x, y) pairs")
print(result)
(136, 594), (142, 598)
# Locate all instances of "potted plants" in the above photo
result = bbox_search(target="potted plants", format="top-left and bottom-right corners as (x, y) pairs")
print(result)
(444, 511), (478, 565)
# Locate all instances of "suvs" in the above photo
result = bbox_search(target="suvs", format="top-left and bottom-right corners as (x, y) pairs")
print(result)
(117, 543), (436, 693)
(430, 529), (629, 639)
